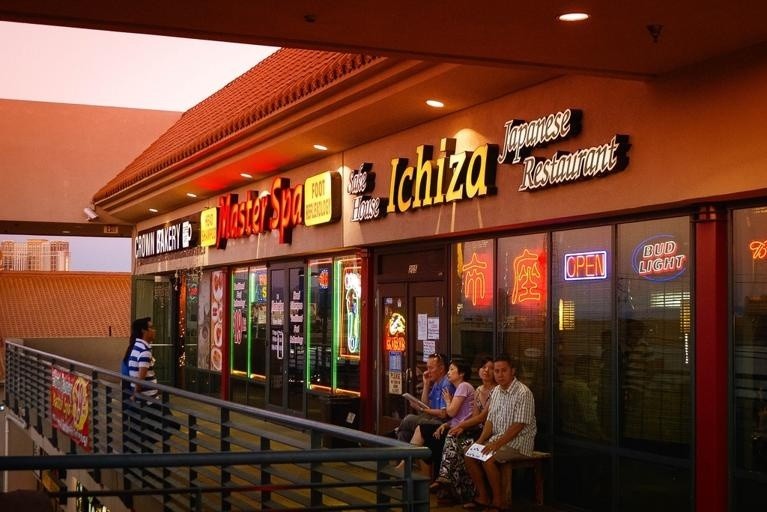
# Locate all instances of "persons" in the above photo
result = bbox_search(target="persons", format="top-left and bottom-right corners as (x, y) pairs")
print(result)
(393, 359), (475, 481)
(460, 354), (537, 511)
(384, 352), (456, 443)
(125, 316), (182, 446)
(538, 313), (658, 437)
(427, 356), (497, 507)
(120, 332), (136, 424)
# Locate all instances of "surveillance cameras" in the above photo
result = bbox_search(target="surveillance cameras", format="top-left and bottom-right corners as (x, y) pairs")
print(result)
(83, 207), (100, 222)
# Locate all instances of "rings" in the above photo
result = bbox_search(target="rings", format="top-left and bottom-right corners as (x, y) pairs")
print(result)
(489, 447), (491, 450)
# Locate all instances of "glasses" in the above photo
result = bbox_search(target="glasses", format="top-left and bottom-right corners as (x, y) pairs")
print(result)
(434, 353), (442, 359)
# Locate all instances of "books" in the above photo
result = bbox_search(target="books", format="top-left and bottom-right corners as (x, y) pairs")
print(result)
(464, 442), (496, 462)
(401, 392), (430, 409)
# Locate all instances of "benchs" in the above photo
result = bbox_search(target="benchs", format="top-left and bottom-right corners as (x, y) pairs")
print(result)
(426, 449), (552, 510)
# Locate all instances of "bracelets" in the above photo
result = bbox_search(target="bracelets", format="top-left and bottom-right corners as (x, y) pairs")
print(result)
(418, 407), (423, 414)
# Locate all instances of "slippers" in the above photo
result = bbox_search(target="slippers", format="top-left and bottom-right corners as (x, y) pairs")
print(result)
(463, 500), (505, 512)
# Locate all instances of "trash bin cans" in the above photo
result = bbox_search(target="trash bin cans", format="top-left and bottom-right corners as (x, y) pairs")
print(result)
(318, 394), (360, 449)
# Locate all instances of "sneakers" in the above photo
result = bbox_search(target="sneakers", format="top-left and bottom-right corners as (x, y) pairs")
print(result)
(382, 429), (398, 439)
(429, 481), (457, 505)
